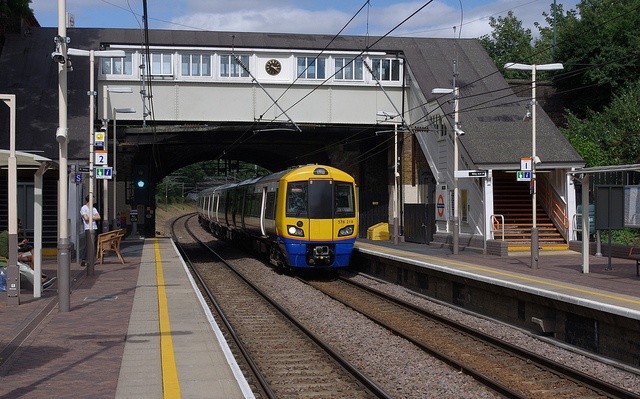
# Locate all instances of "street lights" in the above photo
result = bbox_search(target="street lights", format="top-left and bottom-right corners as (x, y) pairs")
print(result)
(112, 107), (136, 229)
(100, 85), (133, 257)
(377, 111), (399, 245)
(67, 47), (126, 275)
(432, 87), (465, 255)
(502, 62), (565, 268)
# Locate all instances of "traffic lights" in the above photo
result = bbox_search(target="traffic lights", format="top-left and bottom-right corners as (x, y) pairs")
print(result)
(135, 177), (147, 190)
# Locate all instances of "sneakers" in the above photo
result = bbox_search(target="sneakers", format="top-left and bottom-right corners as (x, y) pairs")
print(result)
(43, 282), (57, 290)
(41, 277), (56, 287)
(80, 261), (85, 266)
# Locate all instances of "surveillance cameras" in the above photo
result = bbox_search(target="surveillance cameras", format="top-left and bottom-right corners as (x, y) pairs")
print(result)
(534, 156), (541, 166)
(456, 129), (465, 136)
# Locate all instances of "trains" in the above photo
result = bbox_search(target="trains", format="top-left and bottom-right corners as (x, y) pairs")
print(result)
(197, 163), (360, 274)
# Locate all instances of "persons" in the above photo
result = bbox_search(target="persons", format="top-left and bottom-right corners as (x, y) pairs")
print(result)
(0, 258), (57, 292)
(79, 194), (102, 267)
(0, 218), (34, 266)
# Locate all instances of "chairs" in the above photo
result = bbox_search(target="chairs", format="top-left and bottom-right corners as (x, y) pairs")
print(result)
(97, 234), (126, 266)
(96, 228), (126, 259)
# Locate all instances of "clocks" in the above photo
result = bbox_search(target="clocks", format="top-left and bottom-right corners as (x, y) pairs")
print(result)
(265, 59), (281, 76)
